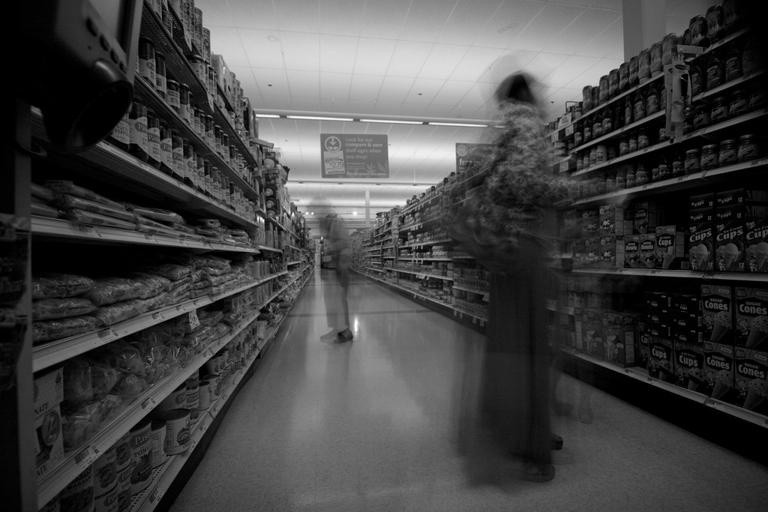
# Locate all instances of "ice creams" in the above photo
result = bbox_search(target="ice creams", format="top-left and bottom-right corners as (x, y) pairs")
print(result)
(687, 242), (767, 410)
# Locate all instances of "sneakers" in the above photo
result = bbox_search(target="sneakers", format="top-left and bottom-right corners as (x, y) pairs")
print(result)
(318, 329), (354, 344)
(463, 425), (565, 493)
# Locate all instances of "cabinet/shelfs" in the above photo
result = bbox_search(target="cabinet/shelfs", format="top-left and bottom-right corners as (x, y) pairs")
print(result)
(0, 2), (768, 511)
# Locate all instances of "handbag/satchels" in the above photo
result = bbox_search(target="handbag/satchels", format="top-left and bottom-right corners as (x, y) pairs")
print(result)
(440, 203), (534, 278)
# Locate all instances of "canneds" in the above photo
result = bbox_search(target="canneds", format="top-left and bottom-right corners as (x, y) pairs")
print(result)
(105, 33), (255, 223)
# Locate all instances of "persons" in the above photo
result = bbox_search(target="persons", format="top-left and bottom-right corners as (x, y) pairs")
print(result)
(317, 206), (354, 346)
(461, 69), (632, 483)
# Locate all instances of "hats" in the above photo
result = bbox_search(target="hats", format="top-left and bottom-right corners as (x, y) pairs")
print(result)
(495, 71), (534, 106)
